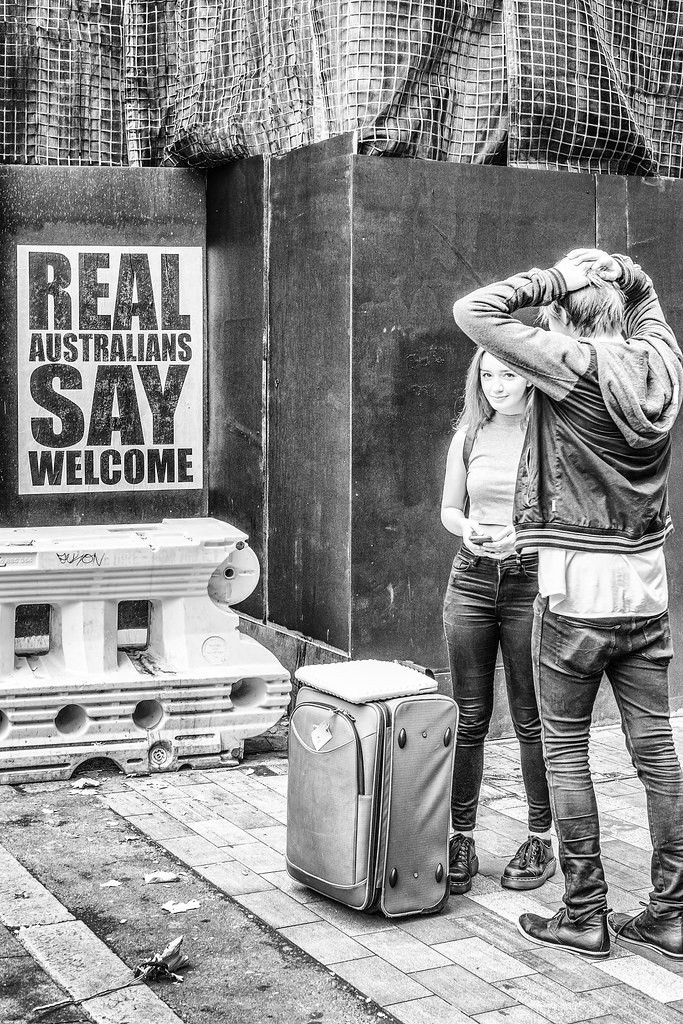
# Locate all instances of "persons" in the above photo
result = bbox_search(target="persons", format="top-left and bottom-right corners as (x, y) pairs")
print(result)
(440, 347), (556, 895)
(452, 249), (683, 960)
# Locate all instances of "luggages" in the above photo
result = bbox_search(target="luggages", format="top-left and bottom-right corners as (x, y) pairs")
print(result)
(288, 661), (459, 919)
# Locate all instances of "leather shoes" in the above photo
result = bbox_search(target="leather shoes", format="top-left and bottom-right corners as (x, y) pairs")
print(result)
(448, 834), (479, 895)
(501, 834), (556, 890)
(606, 895), (683, 962)
(517, 902), (610, 957)
